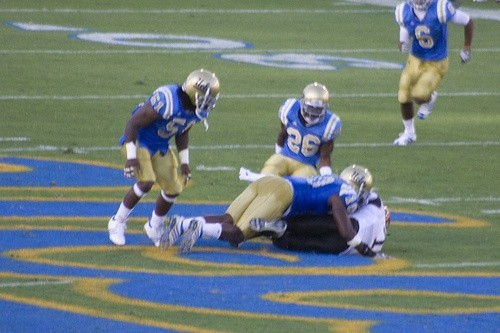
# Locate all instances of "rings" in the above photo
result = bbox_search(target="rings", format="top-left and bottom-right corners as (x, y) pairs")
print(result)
(124, 167), (135, 178)
(185, 177), (188, 181)
(188, 173), (191, 177)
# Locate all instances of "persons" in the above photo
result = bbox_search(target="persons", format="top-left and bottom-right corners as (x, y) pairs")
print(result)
(394, 0), (475, 148)
(248, 192), (389, 257)
(260, 83), (341, 176)
(159, 165), (372, 254)
(107, 69), (221, 245)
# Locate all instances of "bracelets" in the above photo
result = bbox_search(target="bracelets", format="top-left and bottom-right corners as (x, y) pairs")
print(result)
(348, 235), (362, 248)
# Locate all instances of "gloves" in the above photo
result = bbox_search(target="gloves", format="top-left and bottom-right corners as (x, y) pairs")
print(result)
(460, 51), (471, 64)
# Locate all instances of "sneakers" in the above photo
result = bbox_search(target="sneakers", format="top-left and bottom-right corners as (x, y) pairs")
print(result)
(249, 218), (288, 238)
(416, 90), (438, 120)
(107, 216), (127, 245)
(143, 221), (164, 247)
(393, 132), (417, 146)
(178, 215), (205, 254)
(160, 214), (187, 252)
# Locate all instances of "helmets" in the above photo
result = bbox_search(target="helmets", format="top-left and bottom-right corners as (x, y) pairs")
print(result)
(408, 0), (432, 10)
(301, 81), (330, 125)
(180, 68), (221, 112)
(339, 164), (374, 200)
